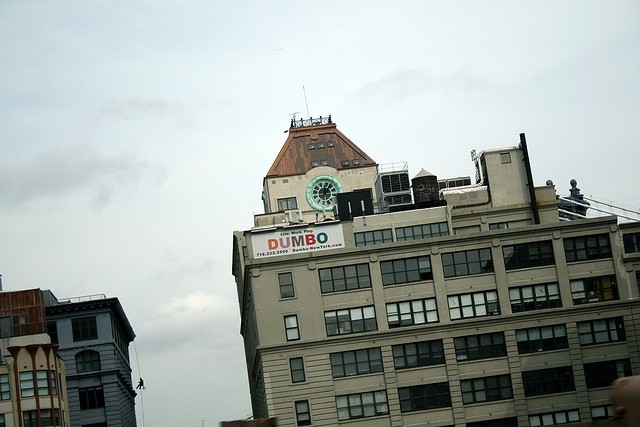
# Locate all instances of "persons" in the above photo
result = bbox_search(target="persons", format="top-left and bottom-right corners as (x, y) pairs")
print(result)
(136, 378), (144, 390)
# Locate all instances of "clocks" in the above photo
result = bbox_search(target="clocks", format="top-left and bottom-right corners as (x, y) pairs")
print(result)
(305, 175), (343, 213)
